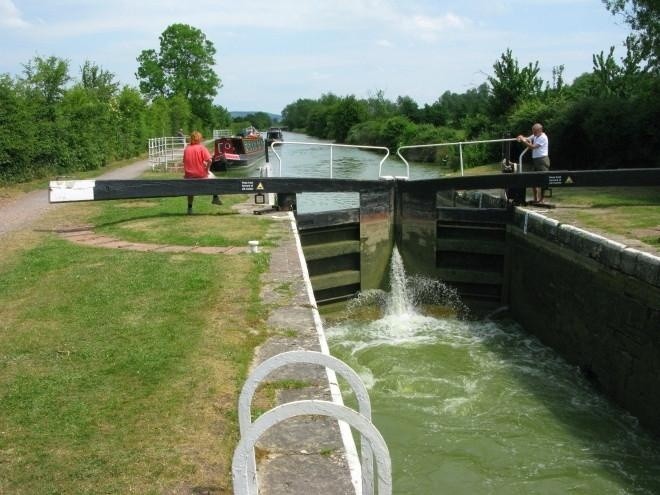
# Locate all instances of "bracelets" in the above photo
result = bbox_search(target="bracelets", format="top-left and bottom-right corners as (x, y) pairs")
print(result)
(523, 140), (527, 142)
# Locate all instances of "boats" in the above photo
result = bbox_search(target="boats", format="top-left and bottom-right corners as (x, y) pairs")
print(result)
(209, 132), (266, 170)
(264, 127), (284, 146)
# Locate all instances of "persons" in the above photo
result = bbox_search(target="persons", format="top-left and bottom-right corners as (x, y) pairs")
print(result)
(178, 128), (184, 142)
(517, 123), (551, 204)
(183, 131), (223, 215)
